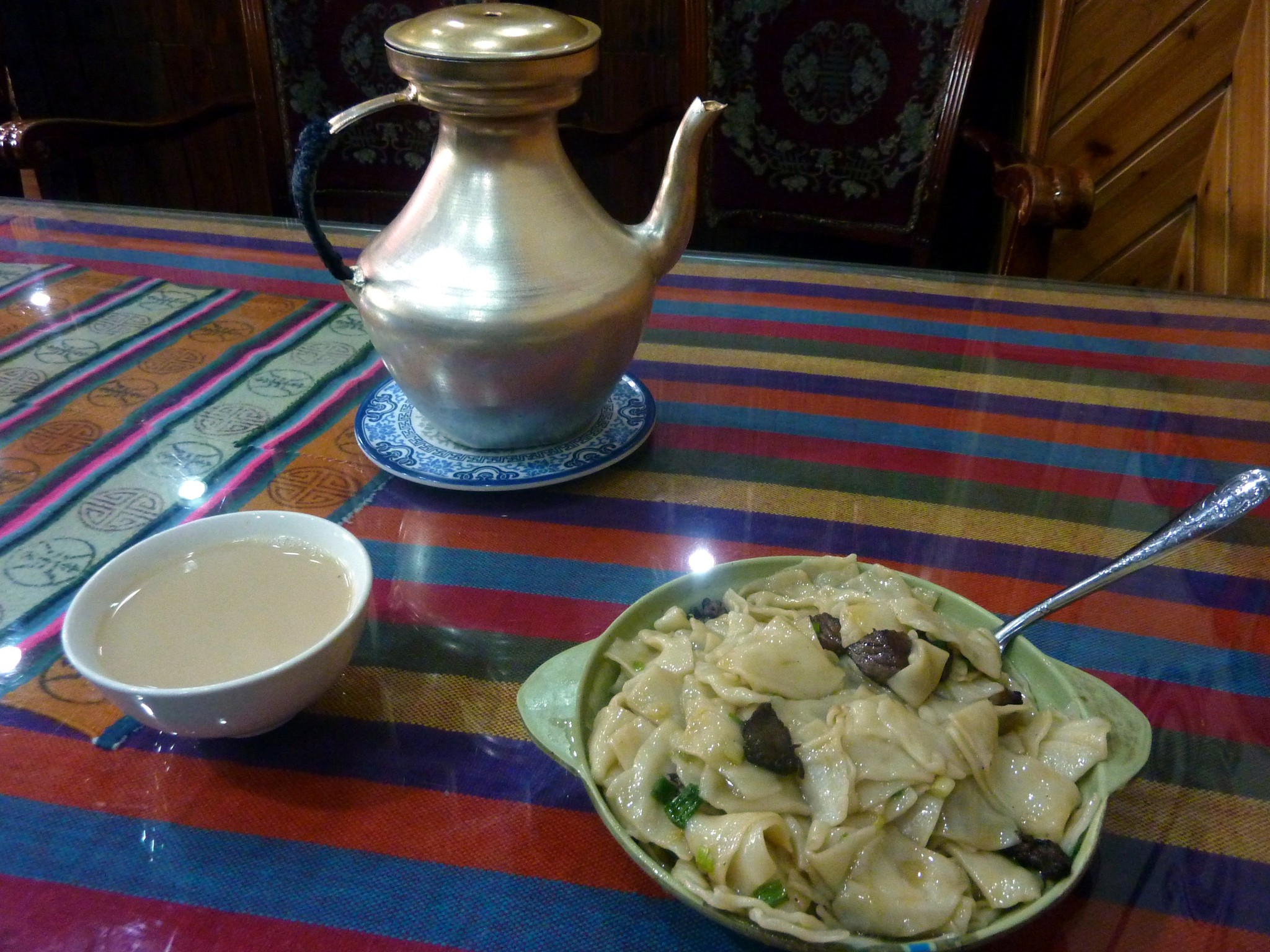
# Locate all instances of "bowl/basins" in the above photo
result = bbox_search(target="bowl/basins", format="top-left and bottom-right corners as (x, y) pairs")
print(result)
(60, 509), (375, 740)
(514, 552), (1153, 952)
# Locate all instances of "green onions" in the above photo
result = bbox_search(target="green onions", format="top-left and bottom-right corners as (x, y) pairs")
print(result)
(631, 620), (955, 907)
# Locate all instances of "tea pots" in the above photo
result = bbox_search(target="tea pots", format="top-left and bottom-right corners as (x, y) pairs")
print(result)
(289, 1), (731, 451)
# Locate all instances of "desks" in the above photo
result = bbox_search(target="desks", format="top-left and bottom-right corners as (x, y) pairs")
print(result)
(0, 191), (1270, 951)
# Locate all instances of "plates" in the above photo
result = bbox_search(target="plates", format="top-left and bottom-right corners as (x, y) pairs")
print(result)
(356, 369), (658, 490)
